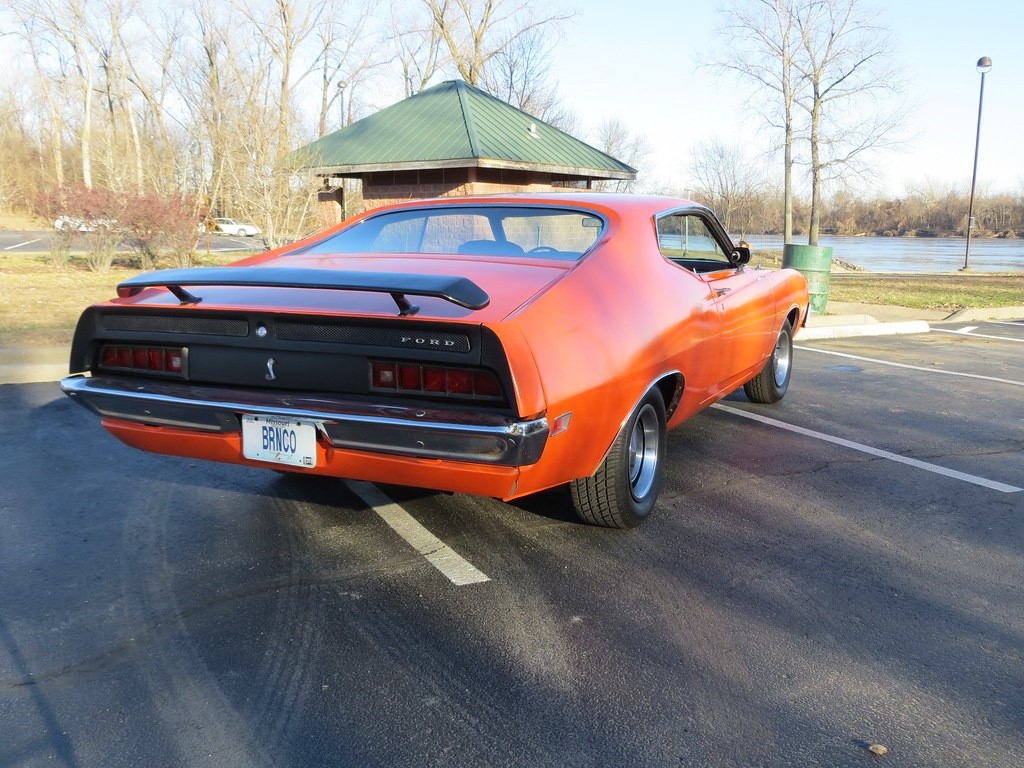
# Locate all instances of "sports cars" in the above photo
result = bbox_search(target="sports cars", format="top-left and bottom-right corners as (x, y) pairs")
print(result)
(58, 192), (810, 529)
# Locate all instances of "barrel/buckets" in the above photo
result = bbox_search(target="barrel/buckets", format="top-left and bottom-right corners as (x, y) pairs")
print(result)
(781, 244), (833, 314)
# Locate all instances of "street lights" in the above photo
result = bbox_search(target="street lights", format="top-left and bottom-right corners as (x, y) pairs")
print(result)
(963, 56), (992, 269)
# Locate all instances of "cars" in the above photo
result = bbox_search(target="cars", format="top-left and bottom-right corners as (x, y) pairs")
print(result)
(198, 218), (261, 237)
(55, 209), (126, 235)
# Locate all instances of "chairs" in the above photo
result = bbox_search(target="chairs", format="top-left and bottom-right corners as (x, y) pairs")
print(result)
(458, 239), (525, 257)
